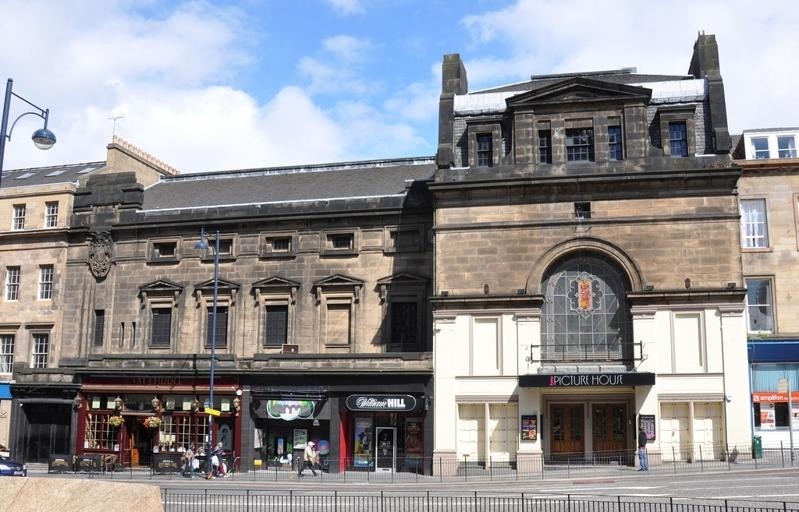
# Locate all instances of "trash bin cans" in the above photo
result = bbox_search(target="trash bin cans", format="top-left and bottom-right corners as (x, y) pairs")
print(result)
(752, 436), (762, 459)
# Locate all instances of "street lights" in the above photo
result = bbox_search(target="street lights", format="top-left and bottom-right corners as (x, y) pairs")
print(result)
(0, 78), (56, 187)
(195, 228), (220, 476)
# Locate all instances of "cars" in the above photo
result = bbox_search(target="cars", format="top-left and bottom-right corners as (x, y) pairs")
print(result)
(0, 454), (27, 478)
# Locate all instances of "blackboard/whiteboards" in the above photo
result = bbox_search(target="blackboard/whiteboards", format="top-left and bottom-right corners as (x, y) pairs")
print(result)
(521, 415), (537, 441)
(638, 414), (656, 441)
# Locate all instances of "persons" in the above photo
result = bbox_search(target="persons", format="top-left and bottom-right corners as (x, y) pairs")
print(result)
(297, 440), (319, 477)
(637, 426), (648, 472)
(191, 446), (206, 471)
(213, 442), (230, 478)
(178, 444), (197, 480)
